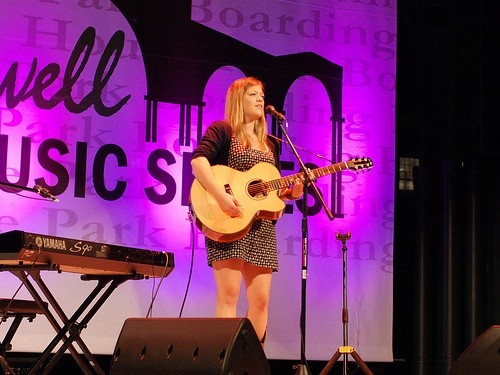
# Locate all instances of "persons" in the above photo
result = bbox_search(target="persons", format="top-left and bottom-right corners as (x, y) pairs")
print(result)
(190, 78), (304, 351)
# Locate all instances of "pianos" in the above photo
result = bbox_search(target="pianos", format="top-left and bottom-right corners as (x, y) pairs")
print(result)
(0, 230), (175, 278)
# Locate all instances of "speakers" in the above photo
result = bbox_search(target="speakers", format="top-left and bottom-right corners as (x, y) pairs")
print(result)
(109, 318), (271, 375)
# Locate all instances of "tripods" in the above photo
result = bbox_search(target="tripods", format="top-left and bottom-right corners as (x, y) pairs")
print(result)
(318, 232), (374, 375)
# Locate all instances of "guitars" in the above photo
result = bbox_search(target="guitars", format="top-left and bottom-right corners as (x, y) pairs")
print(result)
(188, 156), (374, 243)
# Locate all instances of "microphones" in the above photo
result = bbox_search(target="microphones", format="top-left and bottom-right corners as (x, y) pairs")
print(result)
(265, 105), (286, 122)
(33, 185), (58, 201)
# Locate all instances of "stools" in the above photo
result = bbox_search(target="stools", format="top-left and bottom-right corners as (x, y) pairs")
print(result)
(0, 297), (48, 375)
